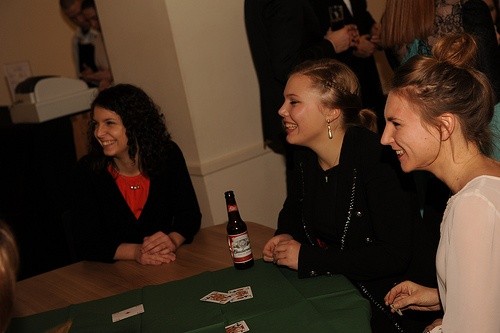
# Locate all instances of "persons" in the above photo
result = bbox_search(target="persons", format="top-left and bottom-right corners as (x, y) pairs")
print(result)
(242, 0), (360, 191)
(380, 0), (500, 112)
(260, 58), (445, 333)
(68, 83), (202, 264)
(325, 0), (386, 133)
(380, 32), (500, 332)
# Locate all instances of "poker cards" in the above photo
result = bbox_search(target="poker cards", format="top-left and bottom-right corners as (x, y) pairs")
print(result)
(200, 284), (254, 305)
(112, 304), (145, 323)
(224, 320), (249, 333)
(47, 318), (74, 333)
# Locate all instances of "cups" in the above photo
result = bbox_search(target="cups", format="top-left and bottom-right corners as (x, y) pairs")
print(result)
(330, 6), (344, 31)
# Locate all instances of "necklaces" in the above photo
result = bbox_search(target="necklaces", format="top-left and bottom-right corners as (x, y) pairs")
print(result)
(447, 151), (483, 197)
(113, 164), (149, 190)
(316, 148), (342, 184)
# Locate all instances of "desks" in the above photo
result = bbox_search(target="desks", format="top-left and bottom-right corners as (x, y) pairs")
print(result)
(5, 220), (373, 333)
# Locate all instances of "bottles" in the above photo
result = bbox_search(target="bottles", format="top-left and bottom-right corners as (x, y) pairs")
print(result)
(224, 191), (254, 270)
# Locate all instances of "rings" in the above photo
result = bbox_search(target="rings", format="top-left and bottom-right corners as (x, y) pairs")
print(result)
(351, 39), (356, 44)
